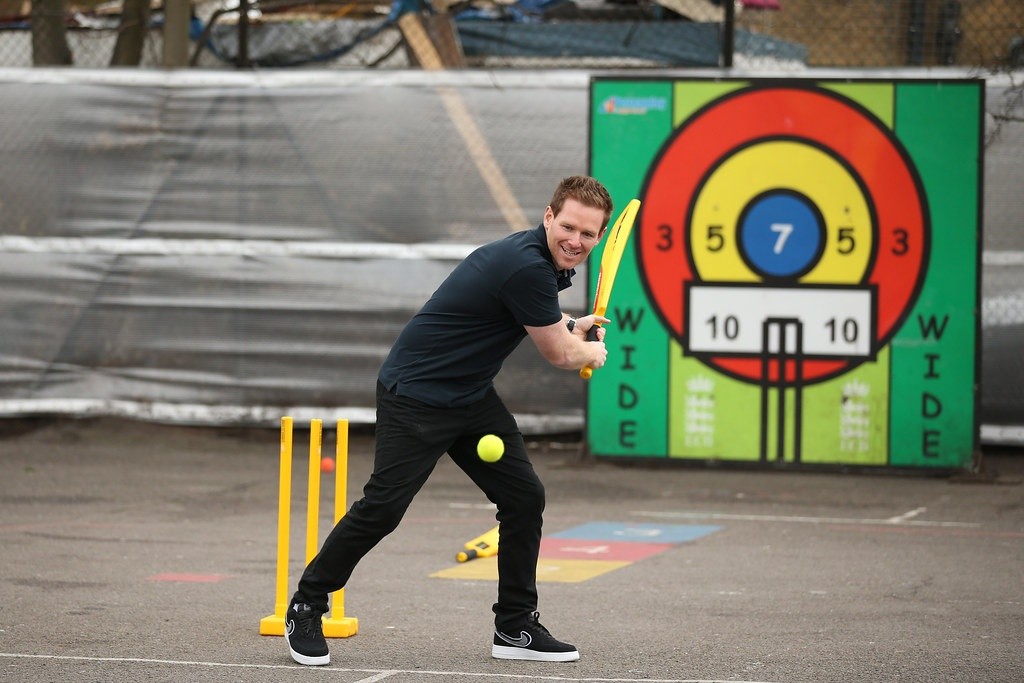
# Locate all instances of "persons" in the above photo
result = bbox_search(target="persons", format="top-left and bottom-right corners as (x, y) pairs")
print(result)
(283, 176), (611, 665)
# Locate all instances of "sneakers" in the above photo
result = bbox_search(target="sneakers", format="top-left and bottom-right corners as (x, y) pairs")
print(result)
(492, 611), (580, 662)
(285, 601), (330, 666)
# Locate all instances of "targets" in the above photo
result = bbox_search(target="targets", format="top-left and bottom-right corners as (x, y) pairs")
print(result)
(583, 80), (988, 468)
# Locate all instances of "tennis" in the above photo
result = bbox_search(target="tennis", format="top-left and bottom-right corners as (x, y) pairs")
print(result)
(478, 433), (504, 462)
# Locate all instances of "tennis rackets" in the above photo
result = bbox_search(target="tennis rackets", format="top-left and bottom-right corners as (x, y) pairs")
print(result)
(580, 197), (640, 378)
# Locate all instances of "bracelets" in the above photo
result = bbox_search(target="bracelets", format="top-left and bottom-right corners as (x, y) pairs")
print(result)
(567, 317), (576, 331)
